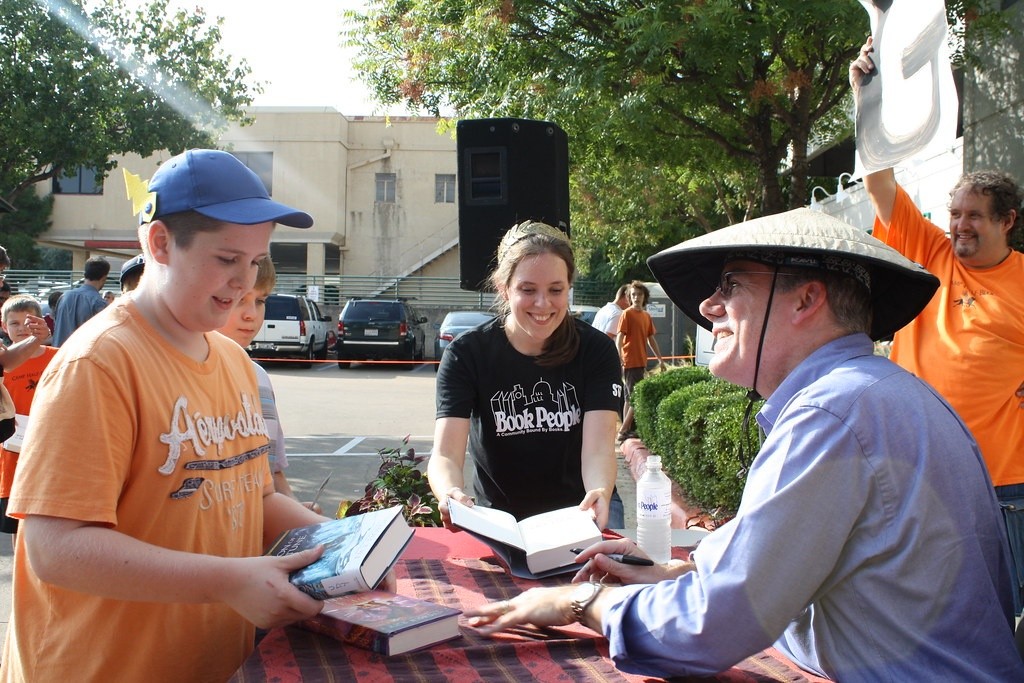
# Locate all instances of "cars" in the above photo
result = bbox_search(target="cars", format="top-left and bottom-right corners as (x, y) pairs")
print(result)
(432, 311), (496, 371)
(568, 304), (603, 327)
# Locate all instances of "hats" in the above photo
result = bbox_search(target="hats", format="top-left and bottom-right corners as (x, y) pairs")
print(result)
(137, 147), (314, 228)
(644, 207), (941, 343)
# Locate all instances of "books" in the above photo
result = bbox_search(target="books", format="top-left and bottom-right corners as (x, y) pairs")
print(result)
(262, 503), (417, 600)
(309, 587), (461, 655)
(3, 413), (29, 454)
(447, 495), (606, 578)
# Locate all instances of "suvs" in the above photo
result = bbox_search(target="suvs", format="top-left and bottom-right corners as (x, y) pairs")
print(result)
(251, 291), (335, 370)
(332, 296), (429, 370)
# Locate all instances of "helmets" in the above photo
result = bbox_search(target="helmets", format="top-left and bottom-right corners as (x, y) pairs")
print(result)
(119, 253), (147, 292)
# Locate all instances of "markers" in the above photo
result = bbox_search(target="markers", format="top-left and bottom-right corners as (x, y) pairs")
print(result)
(569, 548), (654, 566)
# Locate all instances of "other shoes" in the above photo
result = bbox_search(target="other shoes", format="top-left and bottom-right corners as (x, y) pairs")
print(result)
(616, 431), (639, 441)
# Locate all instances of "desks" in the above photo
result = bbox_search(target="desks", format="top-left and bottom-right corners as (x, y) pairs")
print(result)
(226, 528), (832, 683)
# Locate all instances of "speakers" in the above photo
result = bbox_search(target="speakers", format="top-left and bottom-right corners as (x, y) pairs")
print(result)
(457, 117), (570, 291)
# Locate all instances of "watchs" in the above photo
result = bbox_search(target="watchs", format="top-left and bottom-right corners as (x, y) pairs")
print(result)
(570, 580), (606, 629)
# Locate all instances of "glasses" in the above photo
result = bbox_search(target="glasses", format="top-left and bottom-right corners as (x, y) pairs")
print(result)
(0, 275), (6, 281)
(720, 271), (799, 296)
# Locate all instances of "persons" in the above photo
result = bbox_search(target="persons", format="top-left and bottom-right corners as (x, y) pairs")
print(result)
(119, 254), (146, 292)
(51, 257), (108, 347)
(44, 291), (63, 336)
(428, 220), (625, 533)
(2, 149), (397, 683)
(0, 246), (59, 553)
(849, 36), (1024, 618)
(591, 281), (667, 441)
(215, 254), (322, 515)
(103, 290), (115, 303)
(463, 208), (1024, 683)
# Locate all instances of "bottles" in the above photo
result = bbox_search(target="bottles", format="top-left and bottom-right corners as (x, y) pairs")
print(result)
(636, 454), (673, 564)
